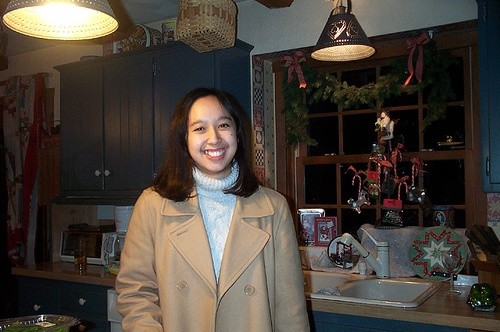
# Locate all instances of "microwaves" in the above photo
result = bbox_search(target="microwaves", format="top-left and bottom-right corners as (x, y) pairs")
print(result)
(58, 230), (103, 268)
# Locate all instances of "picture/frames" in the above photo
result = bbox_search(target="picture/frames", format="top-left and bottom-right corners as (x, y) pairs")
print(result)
(297, 209), (337, 247)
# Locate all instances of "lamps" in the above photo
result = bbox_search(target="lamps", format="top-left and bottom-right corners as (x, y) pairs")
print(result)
(310, 0), (377, 62)
(0, 0), (120, 41)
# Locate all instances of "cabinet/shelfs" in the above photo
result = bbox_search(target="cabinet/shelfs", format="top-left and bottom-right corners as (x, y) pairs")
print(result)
(12, 275), (111, 332)
(53, 38), (255, 197)
(476, 0), (500, 193)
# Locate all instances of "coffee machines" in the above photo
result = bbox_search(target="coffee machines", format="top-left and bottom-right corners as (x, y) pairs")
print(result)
(114, 206), (133, 234)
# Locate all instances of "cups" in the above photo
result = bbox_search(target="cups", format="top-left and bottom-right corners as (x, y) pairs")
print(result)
(74, 236), (87, 271)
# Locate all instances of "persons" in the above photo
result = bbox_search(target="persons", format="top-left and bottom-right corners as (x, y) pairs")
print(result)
(115, 88), (310, 332)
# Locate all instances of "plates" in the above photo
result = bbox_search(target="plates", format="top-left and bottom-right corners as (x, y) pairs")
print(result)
(409, 225), (468, 282)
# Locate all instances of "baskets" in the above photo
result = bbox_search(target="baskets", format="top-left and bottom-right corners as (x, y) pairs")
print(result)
(176, 0), (237, 54)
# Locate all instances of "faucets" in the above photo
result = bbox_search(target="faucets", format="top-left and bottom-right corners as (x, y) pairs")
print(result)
(339, 232), (389, 279)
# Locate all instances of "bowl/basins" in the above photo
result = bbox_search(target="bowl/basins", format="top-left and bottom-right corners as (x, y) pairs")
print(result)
(0, 313), (81, 332)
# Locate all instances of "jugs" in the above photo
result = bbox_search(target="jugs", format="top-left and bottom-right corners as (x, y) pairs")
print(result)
(102, 233), (124, 262)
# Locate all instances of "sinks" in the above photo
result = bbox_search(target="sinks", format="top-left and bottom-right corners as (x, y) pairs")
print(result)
(334, 279), (443, 308)
(302, 269), (355, 294)
